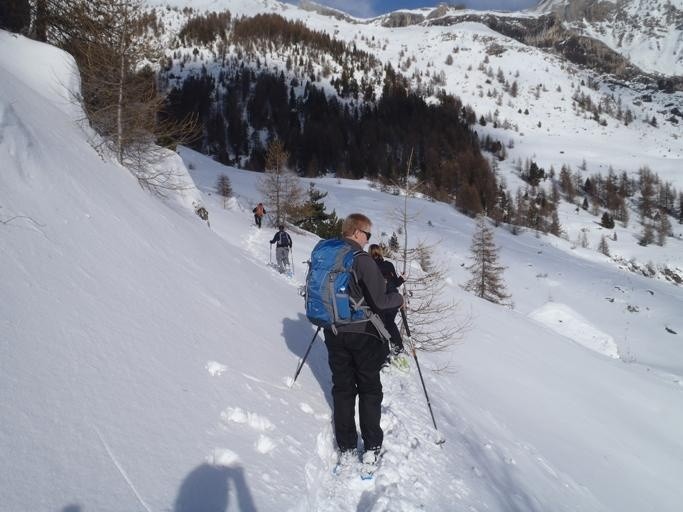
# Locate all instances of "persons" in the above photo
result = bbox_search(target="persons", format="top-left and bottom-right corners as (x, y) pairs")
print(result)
(365, 244), (405, 355)
(268, 225), (293, 275)
(252, 203), (265, 229)
(302, 213), (408, 465)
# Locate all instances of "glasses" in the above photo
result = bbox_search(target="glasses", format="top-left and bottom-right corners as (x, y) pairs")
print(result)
(359, 229), (371, 241)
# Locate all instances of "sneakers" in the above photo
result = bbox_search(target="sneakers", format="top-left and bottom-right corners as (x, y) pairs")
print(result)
(381, 343), (410, 367)
(339, 446), (385, 466)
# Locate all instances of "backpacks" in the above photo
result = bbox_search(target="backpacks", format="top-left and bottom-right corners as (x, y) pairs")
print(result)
(304, 240), (369, 329)
(256, 205), (263, 217)
(277, 232), (290, 245)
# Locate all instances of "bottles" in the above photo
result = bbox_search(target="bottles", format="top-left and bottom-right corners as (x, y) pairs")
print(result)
(338, 286), (348, 293)
